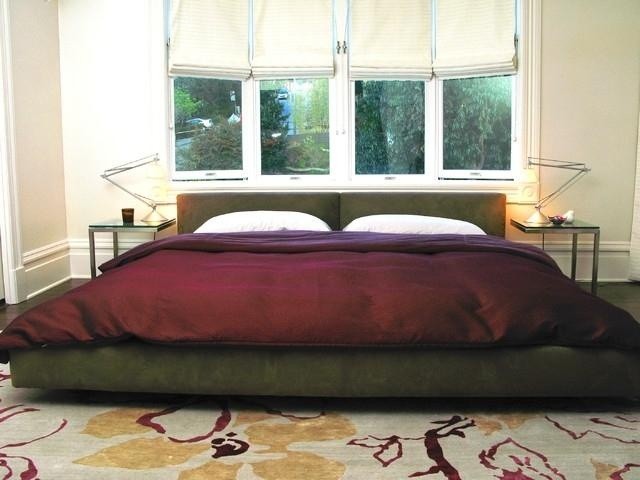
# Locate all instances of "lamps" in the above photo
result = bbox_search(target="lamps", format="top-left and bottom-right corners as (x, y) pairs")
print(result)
(518, 157), (590, 222)
(101, 152), (168, 222)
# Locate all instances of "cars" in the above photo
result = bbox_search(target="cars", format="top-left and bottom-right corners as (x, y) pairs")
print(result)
(176, 116), (213, 136)
(277, 88), (288, 100)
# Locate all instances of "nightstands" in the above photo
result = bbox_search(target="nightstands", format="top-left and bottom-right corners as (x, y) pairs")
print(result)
(88, 218), (176, 280)
(511, 218), (599, 295)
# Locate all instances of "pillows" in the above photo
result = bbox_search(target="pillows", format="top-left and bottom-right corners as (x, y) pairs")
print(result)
(194, 211), (332, 232)
(343, 213), (486, 235)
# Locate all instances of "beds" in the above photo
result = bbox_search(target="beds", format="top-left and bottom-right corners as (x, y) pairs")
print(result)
(0, 192), (640, 400)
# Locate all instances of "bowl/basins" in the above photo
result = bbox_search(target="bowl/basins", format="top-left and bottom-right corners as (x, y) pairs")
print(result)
(549, 215), (567, 225)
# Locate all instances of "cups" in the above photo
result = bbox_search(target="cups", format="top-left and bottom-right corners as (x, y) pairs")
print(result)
(121, 207), (134, 226)
